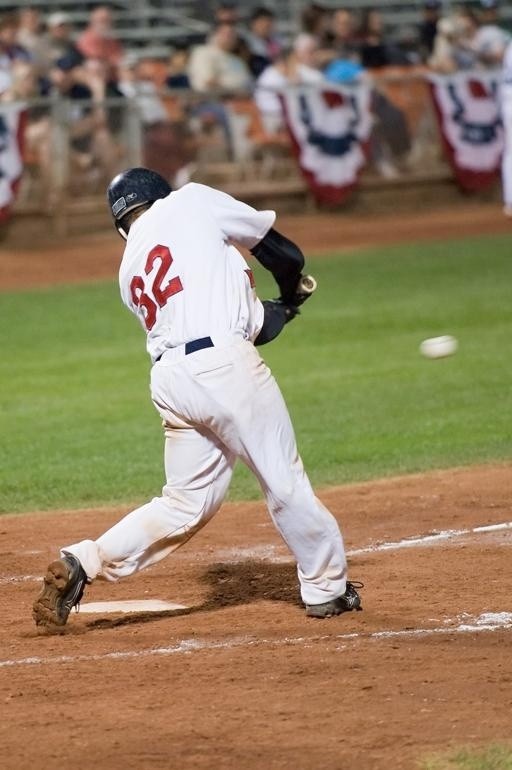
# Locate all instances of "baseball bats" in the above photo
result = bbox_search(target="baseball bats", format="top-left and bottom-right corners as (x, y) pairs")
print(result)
(282, 271), (317, 321)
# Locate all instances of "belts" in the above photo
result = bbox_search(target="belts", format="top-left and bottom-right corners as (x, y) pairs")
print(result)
(154, 336), (214, 362)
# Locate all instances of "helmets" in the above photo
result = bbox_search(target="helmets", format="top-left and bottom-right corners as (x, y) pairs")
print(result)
(106, 166), (172, 239)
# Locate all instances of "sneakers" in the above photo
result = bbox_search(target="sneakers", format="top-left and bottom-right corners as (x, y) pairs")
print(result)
(303, 577), (363, 619)
(32, 550), (86, 630)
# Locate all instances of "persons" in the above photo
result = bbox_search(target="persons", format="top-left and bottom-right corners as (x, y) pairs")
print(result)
(1, 1), (511, 198)
(31, 164), (366, 625)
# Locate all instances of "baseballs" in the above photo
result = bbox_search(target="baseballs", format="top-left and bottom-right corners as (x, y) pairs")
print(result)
(421, 335), (458, 359)
